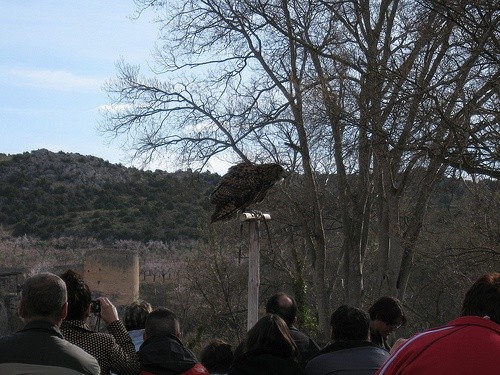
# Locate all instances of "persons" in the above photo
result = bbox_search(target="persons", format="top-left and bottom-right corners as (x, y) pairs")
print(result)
(0, 273), (101, 375)
(59, 269), (141, 375)
(377, 270), (500, 375)
(121, 293), (408, 375)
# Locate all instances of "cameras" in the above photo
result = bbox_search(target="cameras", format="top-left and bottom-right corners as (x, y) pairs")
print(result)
(91, 301), (101, 313)
